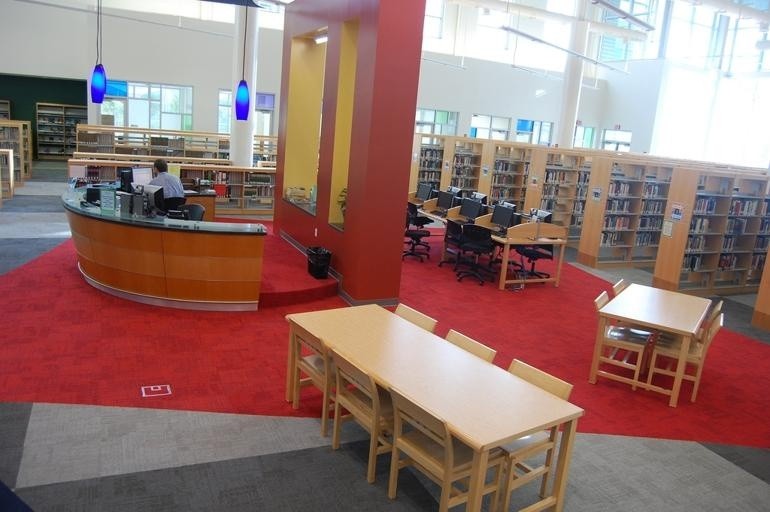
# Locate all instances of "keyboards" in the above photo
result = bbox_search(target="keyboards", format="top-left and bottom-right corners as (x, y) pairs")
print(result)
(491, 230), (505, 236)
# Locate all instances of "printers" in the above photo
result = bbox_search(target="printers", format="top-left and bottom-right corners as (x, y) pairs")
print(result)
(86, 183), (116, 202)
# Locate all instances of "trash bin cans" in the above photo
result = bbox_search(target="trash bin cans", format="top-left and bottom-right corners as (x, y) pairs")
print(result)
(305, 247), (332, 279)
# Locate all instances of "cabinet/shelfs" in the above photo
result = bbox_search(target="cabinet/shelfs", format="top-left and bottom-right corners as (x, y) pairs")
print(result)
(35, 102), (87, 162)
(1, 99), (31, 207)
(68, 124), (278, 214)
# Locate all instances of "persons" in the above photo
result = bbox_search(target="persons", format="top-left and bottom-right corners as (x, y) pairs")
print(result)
(418, 146), (444, 190)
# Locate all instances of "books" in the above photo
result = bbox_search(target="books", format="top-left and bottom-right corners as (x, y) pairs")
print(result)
(492, 153), (518, 199)
(450, 145), (477, 188)
(539, 161), (565, 223)
(570, 165), (591, 225)
(600, 167), (665, 246)
(0, 109), (278, 210)
(680, 197), (769, 270)
(520, 163), (530, 200)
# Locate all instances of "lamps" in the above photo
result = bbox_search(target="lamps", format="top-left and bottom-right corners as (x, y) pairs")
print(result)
(314, 34), (329, 45)
(235, 0), (248, 120)
(91, 1), (107, 104)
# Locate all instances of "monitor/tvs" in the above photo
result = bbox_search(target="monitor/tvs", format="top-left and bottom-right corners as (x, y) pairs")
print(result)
(449, 186), (462, 203)
(490, 205), (514, 232)
(437, 191), (454, 212)
(460, 199), (482, 223)
(504, 201), (517, 213)
(415, 183), (433, 201)
(130, 183), (165, 212)
(536, 210), (552, 223)
(472, 192), (487, 205)
(131, 167), (153, 185)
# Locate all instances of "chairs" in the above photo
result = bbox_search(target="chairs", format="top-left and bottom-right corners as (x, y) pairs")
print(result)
(403, 221), (430, 263)
(590, 278), (725, 405)
